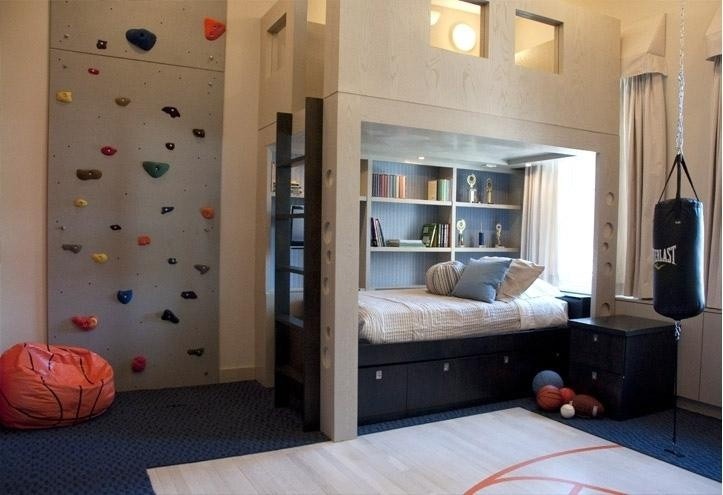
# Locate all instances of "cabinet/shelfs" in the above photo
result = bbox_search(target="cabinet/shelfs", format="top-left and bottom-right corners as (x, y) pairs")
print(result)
(272, 160), (521, 291)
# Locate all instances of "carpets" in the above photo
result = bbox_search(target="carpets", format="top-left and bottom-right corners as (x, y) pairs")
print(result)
(145, 406), (722, 495)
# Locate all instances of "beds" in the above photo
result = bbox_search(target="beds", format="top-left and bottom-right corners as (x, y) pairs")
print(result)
(272, 282), (591, 433)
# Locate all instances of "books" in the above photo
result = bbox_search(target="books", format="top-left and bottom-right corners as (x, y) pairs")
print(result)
(387, 239), (423, 247)
(422, 223), (449, 246)
(374, 174), (406, 199)
(371, 218), (389, 247)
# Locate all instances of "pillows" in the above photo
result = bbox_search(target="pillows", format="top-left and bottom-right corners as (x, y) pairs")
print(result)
(426, 261), (464, 296)
(496, 259), (545, 303)
(516, 278), (564, 300)
(452, 258), (512, 303)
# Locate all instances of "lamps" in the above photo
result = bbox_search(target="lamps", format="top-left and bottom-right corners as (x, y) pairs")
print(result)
(452, 23), (476, 52)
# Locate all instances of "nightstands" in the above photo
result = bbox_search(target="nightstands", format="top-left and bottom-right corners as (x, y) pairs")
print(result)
(566, 314), (679, 421)
